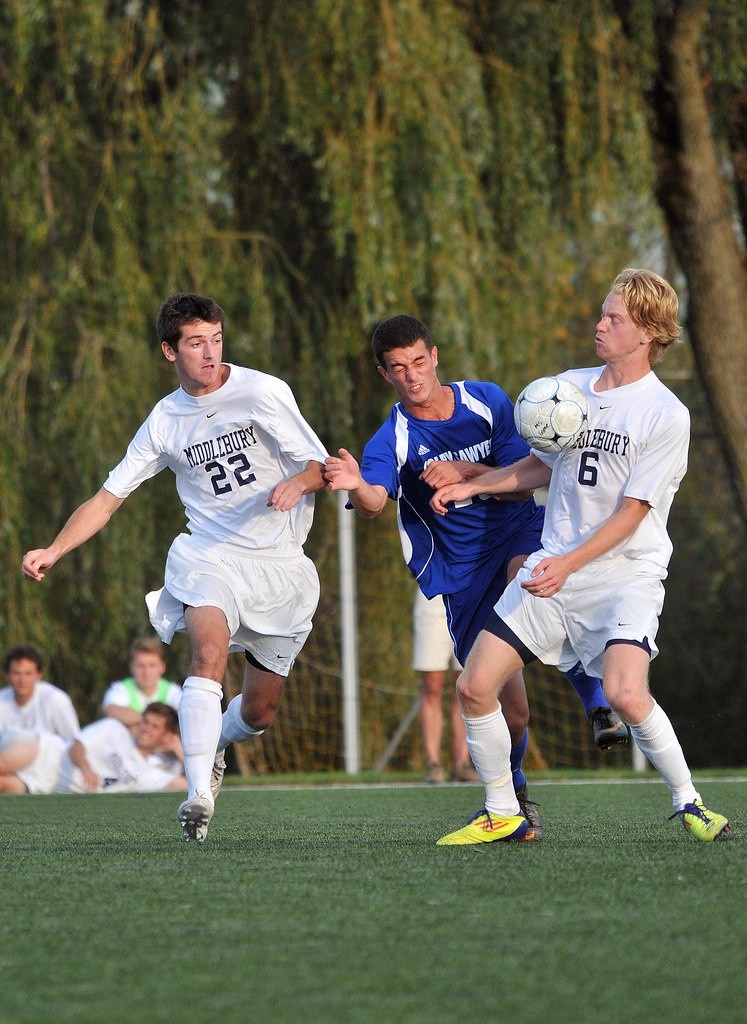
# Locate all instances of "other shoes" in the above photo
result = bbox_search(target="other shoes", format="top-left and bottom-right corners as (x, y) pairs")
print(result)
(426, 762), (445, 782)
(451, 763), (481, 781)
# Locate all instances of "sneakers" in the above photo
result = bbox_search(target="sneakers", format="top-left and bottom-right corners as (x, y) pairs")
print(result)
(177, 788), (214, 845)
(589, 706), (629, 749)
(669, 798), (732, 842)
(516, 780), (544, 841)
(211, 749), (226, 800)
(435, 805), (529, 845)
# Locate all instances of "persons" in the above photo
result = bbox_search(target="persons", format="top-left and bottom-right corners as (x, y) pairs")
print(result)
(437, 269), (731, 845)
(103, 639), (184, 776)
(325, 315), (628, 841)
(413, 586), (480, 783)
(0, 645), (81, 738)
(21, 293), (331, 841)
(0, 702), (186, 795)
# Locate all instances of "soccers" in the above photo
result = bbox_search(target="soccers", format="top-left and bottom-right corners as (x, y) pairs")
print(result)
(512, 375), (590, 455)
